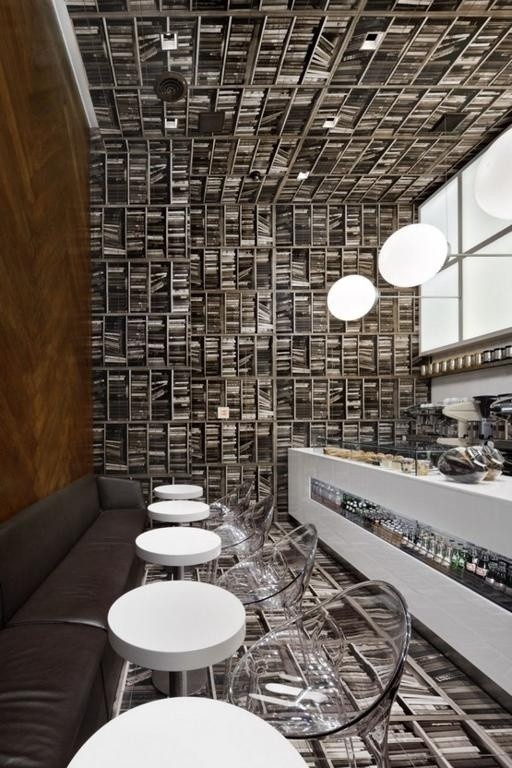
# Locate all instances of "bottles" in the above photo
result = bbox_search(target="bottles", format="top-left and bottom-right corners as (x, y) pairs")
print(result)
(311, 480), (511, 604)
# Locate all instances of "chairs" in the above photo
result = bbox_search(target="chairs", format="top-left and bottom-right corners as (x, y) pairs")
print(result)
(216, 522), (346, 673)
(206, 478), (257, 535)
(214, 493), (288, 584)
(224, 579), (413, 766)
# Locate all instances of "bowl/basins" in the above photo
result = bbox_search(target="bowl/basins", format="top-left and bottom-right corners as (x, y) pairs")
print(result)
(440, 470), (488, 483)
(485, 468), (502, 482)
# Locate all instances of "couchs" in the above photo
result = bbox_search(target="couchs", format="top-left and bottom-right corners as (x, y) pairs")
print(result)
(0, 473), (148, 768)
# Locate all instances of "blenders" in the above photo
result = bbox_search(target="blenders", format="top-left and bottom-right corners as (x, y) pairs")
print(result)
(436, 400), (482, 447)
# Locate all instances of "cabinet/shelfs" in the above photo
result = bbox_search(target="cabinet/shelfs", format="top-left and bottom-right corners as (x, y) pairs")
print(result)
(419, 326), (512, 450)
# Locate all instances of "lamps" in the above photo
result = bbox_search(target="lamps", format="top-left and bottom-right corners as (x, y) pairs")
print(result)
(473, 127), (512, 221)
(376, 223), (512, 288)
(327, 274), (460, 322)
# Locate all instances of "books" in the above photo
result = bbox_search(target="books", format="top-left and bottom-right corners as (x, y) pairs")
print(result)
(64, 1), (512, 522)
(116, 529), (511, 766)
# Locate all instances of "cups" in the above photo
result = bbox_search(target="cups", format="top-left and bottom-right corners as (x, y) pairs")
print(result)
(400, 457), (429, 475)
